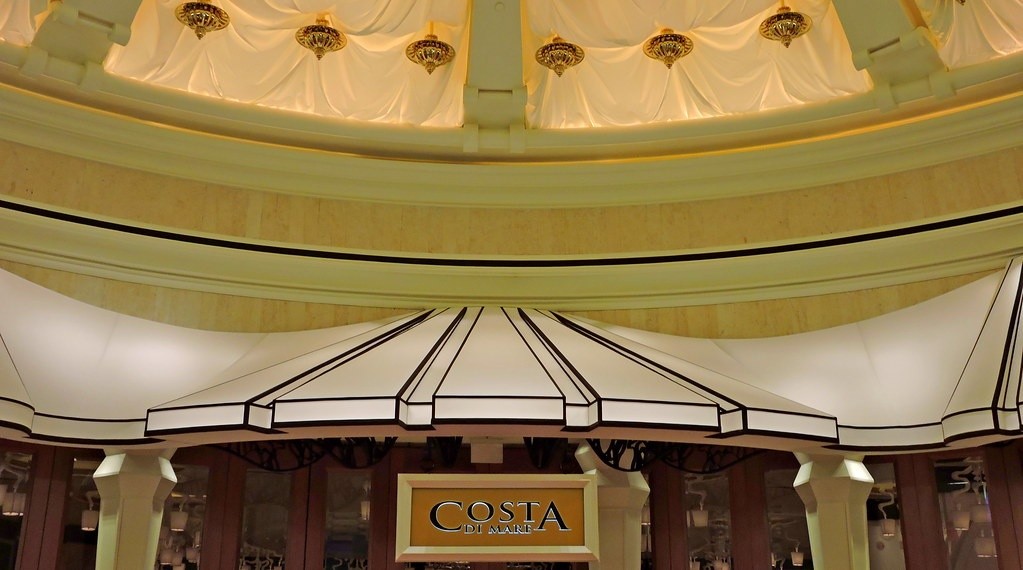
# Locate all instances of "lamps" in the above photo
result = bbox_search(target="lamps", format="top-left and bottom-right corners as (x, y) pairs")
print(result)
(175, 0), (230, 40)
(295, 14), (348, 60)
(405, 19), (456, 75)
(643, 27), (694, 70)
(536, 33), (586, 77)
(0, 464), (998, 570)
(759, 0), (813, 49)
